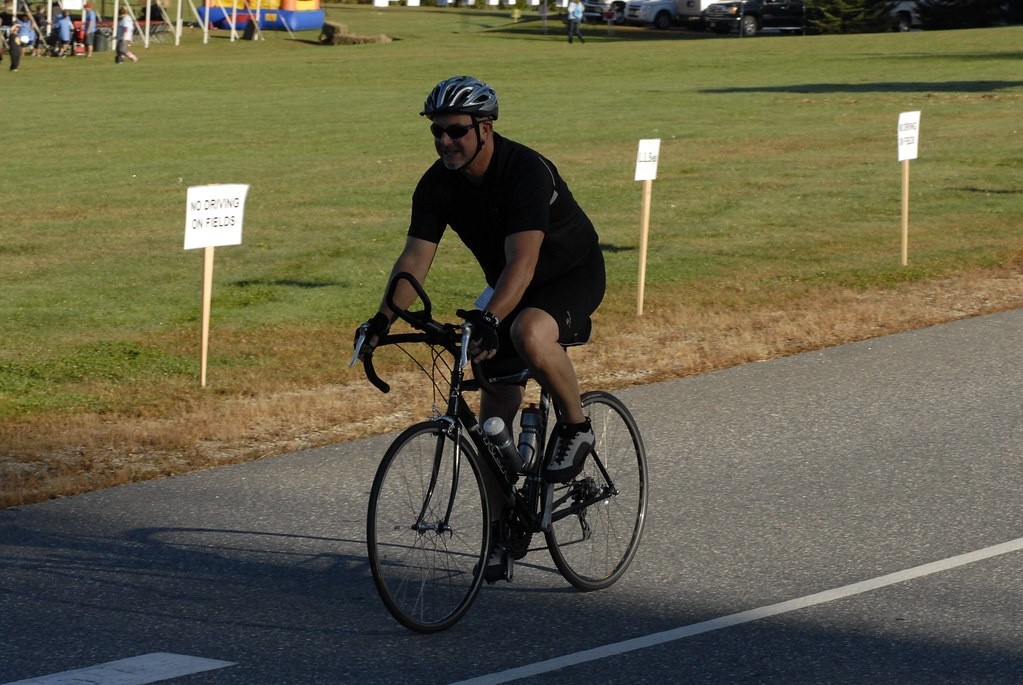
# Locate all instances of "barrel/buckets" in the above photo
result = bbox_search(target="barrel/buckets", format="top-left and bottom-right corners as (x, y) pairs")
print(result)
(74, 29), (86, 43)
(94, 32), (109, 52)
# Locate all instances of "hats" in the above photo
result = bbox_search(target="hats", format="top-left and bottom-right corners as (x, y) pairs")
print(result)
(83, 2), (91, 8)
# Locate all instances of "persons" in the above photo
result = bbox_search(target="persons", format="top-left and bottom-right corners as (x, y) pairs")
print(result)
(51, 9), (75, 58)
(31, 5), (50, 56)
(83, 2), (97, 58)
(355, 75), (607, 585)
(0, 28), (9, 61)
(566, 0), (588, 45)
(14, 14), (37, 47)
(115, 5), (139, 63)
(9, 23), (23, 72)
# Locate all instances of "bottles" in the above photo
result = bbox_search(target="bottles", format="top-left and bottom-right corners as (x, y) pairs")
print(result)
(483, 417), (526, 472)
(518, 403), (543, 474)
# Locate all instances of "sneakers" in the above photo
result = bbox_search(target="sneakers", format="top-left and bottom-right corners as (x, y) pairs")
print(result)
(545, 417), (597, 482)
(472, 519), (511, 580)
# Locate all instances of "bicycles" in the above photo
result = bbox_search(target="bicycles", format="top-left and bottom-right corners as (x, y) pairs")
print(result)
(349, 271), (650, 634)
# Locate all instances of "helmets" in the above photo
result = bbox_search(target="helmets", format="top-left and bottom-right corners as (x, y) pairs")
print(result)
(419, 75), (499, 120)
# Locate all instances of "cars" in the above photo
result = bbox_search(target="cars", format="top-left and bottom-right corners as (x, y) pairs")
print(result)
(703, 0), (819, 36)
(888, 1), (922, 31)
(581, 0), (746, 32)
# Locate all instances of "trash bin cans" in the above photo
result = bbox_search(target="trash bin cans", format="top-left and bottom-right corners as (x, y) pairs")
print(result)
(94, 29), (109, 52)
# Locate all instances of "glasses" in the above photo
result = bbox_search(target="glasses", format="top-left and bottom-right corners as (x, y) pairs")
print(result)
(430, 119), (488, 138)
